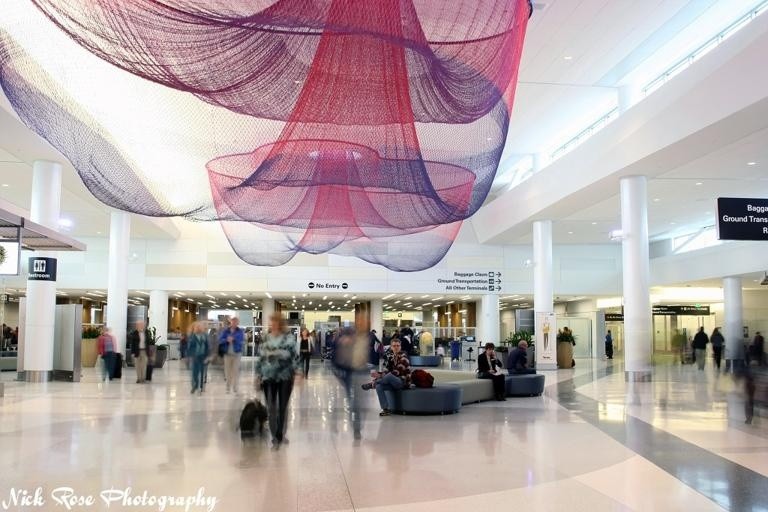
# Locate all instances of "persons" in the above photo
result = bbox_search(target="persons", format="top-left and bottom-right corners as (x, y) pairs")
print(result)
(542, 317), (551, 358)
(0, 323), (18, 351)
(560, 326), (576, 368)
(671, 325), (768, 371)
(96, 311), (445, 455)
(507, 339), (536, 374)
(604, 329), (614, 359)
(478, 342), (506, 401)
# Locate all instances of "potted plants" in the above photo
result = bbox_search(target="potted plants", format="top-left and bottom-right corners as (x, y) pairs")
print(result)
(557, 331), (579, 369)
(80, 326), (102, 369)
(148, 326), (171, 370)
(508, 329), (536, 367)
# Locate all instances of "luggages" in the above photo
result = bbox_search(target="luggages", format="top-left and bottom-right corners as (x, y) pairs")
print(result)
(112, 352), (122, 378)
(239, 399), (267, 438)
(146, 364), (153, 380)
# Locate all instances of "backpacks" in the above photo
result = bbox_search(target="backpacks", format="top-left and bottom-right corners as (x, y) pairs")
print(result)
(412, 368), (435, 388)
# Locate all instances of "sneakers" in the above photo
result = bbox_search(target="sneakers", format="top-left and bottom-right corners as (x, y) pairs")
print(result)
(380, 408), (390, 416)
(362, 383), (373, 390)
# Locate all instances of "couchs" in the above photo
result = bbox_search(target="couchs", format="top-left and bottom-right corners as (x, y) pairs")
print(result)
(374, 346), (546, 415)
(1, 350), (17, 371)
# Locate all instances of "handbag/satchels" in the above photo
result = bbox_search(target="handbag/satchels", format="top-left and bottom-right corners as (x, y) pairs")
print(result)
(217, 342), (226, 356)
